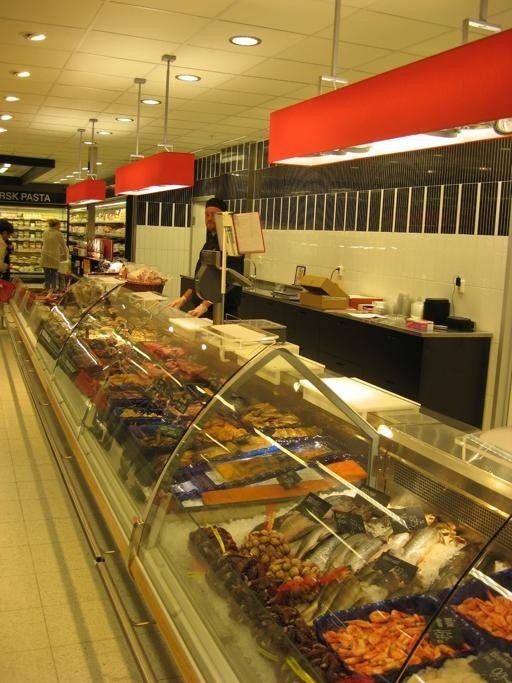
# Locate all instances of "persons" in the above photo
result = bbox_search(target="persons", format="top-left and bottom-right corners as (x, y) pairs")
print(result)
(39, 218), (66, 290)
(166, 198), (244, 321)
(0, 219), (14, 280)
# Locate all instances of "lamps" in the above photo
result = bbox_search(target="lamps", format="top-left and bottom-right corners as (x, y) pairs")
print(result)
(115, 55), (194, 195)
(65, 119), (105, 206)
(268, 0), (512, 167)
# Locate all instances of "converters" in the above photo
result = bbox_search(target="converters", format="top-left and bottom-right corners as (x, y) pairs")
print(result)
(456, 277), (460, 285)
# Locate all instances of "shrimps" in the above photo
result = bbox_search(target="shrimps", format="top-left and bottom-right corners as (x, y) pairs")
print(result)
(453, 590), (512, 642)
(322, 608), (473, 675)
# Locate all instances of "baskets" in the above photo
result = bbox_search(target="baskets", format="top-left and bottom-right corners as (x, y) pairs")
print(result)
(0, 280), (16, 301)
(125, 280), (163, 293)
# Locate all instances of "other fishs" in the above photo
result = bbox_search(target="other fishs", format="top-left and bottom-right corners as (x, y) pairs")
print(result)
(279, 493), (488, 624)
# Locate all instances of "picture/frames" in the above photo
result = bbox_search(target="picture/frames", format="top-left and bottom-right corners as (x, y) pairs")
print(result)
(294, 266), (306, 283)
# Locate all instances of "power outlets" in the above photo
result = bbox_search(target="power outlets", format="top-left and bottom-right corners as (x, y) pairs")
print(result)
(337, 266), (342, 276)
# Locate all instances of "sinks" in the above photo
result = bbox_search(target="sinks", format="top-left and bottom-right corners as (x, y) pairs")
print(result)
(252, 278), (303, 294)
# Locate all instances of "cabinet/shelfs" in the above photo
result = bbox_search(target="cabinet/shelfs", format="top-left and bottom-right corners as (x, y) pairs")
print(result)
(37, 275), (512, 683)
(0, 187), (132, 284)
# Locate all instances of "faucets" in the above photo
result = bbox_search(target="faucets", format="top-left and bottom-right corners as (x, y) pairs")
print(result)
(244, 257), (257, 278)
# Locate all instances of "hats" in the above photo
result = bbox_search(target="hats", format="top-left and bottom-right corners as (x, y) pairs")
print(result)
(207, 198), (227, 212)
